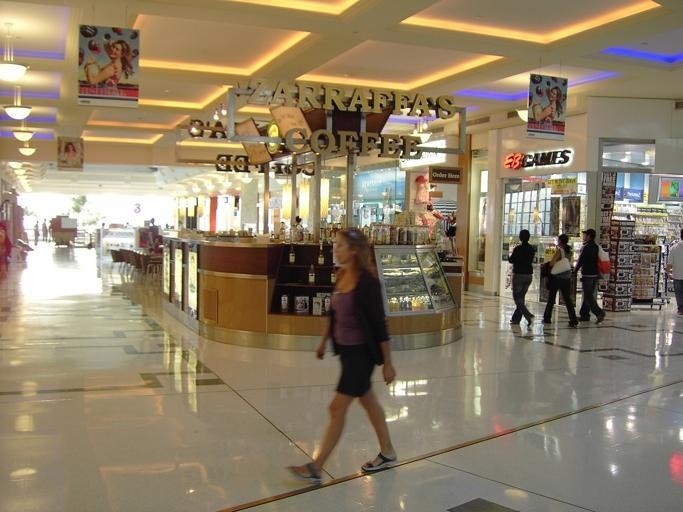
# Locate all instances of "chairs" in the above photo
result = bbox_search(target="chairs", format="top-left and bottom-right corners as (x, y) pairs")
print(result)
(110, 248), (162, 280)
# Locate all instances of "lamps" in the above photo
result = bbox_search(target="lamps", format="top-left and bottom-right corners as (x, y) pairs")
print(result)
(10, 120), (36, 143)
(0, 22), (30, 86)
(18, 143), (37, 157)
(3, 86), (33, 120)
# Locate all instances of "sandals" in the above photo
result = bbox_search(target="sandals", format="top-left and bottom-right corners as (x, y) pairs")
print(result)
(284, 462), (322, 485)
(361, 453), (397, 471)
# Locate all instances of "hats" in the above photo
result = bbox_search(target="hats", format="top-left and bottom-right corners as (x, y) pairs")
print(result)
(581, 228), (595, 238)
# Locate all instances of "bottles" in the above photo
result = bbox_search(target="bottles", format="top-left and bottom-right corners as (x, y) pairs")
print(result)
(407, 298), (412, 311)
(403, 298), (407, 311)
(389, 298), (395, 312)
(399, 297), (403, 311)
(419, 302), (424, 310)
(412, 297), (416, 311)
(416, 225), (424, 244)
(393, 298), (399, 311)
(390, 225), (398, 245)
(320, 218), (343, 243)
(279, 222), (285, 241)
(428, 301), (431, 310)
(398, 224), (408, 244)
(424, 302), (428, 309)
(330, 264), (337, 285)
(290, 224), (297, 244)
(288, 243), (295, 265)
(423, 225), (429, 244)
(415, 298), (419, 310)
(407, 226), (414, 245)
(318, 242), (324, 265)
(302, 227), (309, 241)
(383, 225), (390, 245)
(309, 264), (315, 285)
(372, 224), (382, 245)
(418, 296), (431, 301)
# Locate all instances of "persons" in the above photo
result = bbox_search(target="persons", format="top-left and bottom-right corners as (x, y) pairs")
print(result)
(667, 228), (683, 316)
(532, 86), (563, 130)
(414, 176), (444, 236)
(64, 142), (76, 161)
(151, 239), (162, 256)
(573, 229), (606, 324)
(34, 221), (40, 245)
(285, 227), (397, 484)
(42, 219), (48, 241)
(542, 234), (579, 327)
(508, 229), (535, 331)
(84, 40), (134, 96)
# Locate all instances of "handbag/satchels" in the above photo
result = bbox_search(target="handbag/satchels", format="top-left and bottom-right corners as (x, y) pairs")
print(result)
(597, 244), (610, 274)
(550, 248), (571, 274)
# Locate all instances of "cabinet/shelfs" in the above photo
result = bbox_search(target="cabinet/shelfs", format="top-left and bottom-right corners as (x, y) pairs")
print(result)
(499, 236), (603, 315)
(612, 202), (683, 252)
(599, 171), (618, 252)
(161, 237), (267, 349)
(373, 244), (463, 351)
(268, 244), (373, 353)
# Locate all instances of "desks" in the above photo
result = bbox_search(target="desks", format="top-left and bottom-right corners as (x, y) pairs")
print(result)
(440, 255), (465, 308)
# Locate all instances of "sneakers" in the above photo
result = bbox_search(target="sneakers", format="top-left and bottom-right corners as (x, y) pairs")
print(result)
(527, 315), (534, 327)
(676, 312), (683, 318)
(509, 319), (519, 324)
